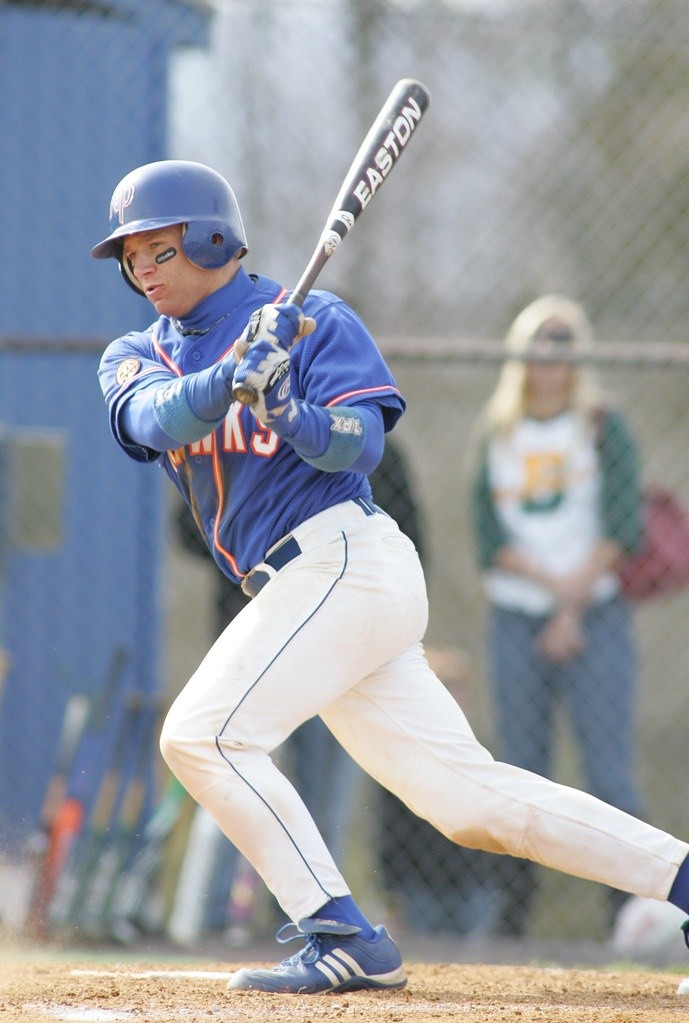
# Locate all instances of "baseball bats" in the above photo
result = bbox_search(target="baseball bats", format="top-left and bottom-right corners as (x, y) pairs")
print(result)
(230, 76), (432, 406)
(19, 688), (297, 956)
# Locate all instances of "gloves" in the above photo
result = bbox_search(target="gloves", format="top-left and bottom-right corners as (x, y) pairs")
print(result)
(222, 303), (317, 397)
(232, 340), (303, 439)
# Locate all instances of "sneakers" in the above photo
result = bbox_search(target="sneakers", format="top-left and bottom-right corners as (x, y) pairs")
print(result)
(227, 917), (407, 992)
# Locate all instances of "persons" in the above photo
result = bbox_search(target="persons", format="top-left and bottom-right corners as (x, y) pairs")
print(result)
(88, 156), (689, 996)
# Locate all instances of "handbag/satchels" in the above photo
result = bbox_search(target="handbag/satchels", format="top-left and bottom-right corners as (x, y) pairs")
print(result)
(616, 489), (689, 600)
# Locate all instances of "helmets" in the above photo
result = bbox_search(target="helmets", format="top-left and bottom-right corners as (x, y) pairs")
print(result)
(91, 160), (249, 297)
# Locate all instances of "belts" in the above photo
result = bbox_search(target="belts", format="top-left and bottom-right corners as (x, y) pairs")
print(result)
(240, 498), (385, 598)
